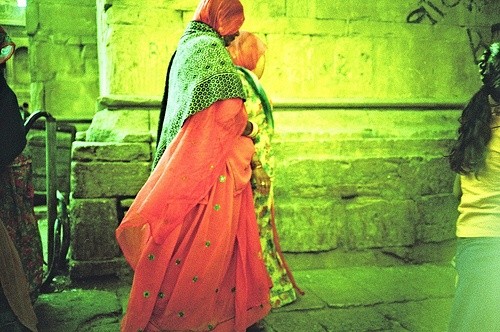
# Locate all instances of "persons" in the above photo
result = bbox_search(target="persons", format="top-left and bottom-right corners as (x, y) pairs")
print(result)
(447, 43), (500, 332)
(0, 28), (46, 331)
(223, 33), (305, 310)
(112, 1), (274, 331)
